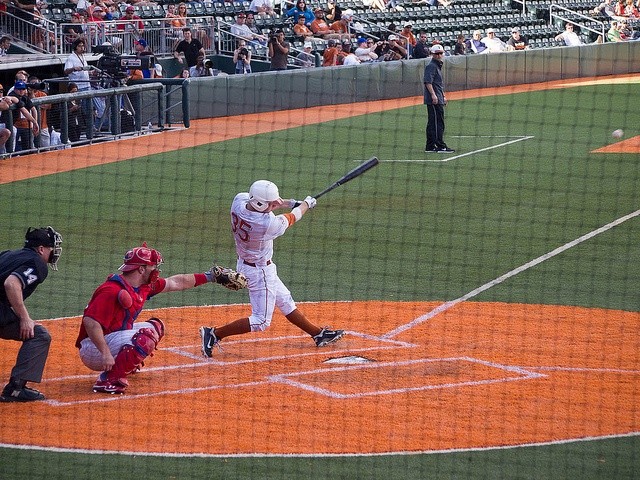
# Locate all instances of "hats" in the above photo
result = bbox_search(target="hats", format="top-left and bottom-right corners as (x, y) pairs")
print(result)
(152, 64), (163, 76)
(134, 38), (146, 47)
(299, 14), (305, 18)
(72, 13), (80, 20)
(404, 22), (412, 27)
(126, 6), (134, 11)
(388, 35), (398, 40)
(304, 42), (312, 48)
(488, 28), (494, 33)
(92, 6), (103, 12)
(315, 8), (323, 13)
(328, 40), (336, 46)
(14, 81), (26, 89)
(358, 38), (367, 43)
(431, 45), (445, 53)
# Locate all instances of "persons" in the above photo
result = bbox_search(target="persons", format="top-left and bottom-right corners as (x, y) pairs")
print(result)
(134, 38), (162, 78)
(293, 14), (313, 38)
(294, 42), (315, 69)
(117, 6), (145, 48)
(0, 36), (11, 57)
(335, 39), (361, 65)
(75, 242), (248, 394)
(164, 2), (187, 27)
(322, 39), (338, 66)
(174, 28), (206, 69)
(230, 12), (266, 43)
(199, 180), (346, 359)
(454, 34), (465, 55)
(424, 44), (455, 154)
(64, 39), (94, 143)
(325, 0), (342, 23)
(189, 51), (209, 76)
(507, 26), (532, 50)
(285, 0), (315, 26)
(415, 31), (429, 58)
(269, 29), (289, 71)
(329, 14), (350, 34)
(60, 83), (82, 148)
(63, 1), (120, 52)
(0, 0), (7, 16)
(0, 70), (51, 160)
(355, 22), (416, 62)
(555, 23), (603, 45)
(91, 75), (112, 134)
(232, 40), (252, 74)
(310, 7), (335, 36)
(173, 69), (190, 78)
(594, 0), (640, 42)
(465, 30), (492, 54)
(481, 28), (515, 53)
(34, 0), (43, 20)
(0, 225), (63, 402)
(361, 0), (452, 13)
(12, 1), (41, 50)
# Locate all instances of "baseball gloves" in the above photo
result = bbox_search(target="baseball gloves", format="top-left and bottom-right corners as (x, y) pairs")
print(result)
(211, 267), (247, 290)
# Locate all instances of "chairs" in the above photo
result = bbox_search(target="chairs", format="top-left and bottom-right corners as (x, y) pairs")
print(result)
(286, 1), (514, 51)
(514, 1), (610, 48)
(36, 0), (298, 40)
(271, 39), (368, 65)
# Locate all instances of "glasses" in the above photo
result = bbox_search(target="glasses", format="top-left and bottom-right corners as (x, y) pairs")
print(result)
(300, 2), (305, 3)
(238, 16), (246, 19)
(180, 7), (185, 9)
(367, 42), (373, 44)
(405, 27), (411, 28)
(434, 52), (444, 55)
(0, 89), (4, 93)
(512, 32), (517, 33)
(420, 36), (426, 38)
(248, 17), (253, 19)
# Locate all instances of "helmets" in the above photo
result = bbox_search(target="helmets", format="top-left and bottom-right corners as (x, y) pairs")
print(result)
(117, 241), (164, 291)
(24, 226), (62, 271)
(250, 179), (284, 212)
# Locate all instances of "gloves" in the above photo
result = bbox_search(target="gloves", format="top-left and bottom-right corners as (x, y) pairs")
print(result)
(291, 200), (303, 209)
(304, 196), (317, 208)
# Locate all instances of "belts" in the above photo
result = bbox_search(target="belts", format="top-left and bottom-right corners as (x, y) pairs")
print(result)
(238, 256), (272, 267)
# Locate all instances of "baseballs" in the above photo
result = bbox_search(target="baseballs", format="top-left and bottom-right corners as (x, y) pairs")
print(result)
(614, 129), (625, 139)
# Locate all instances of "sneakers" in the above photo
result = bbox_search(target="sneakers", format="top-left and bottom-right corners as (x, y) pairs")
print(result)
(425, 147), (437, 152)
(0, 383), (44, 402)
(200, 326), (221, 359)
(311, 327), (345, 347)
(438, 147), (455, 153)
(92, 381), (126, 394)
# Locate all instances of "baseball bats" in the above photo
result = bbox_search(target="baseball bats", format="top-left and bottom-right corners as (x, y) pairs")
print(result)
(315, 156), (379, 198)
